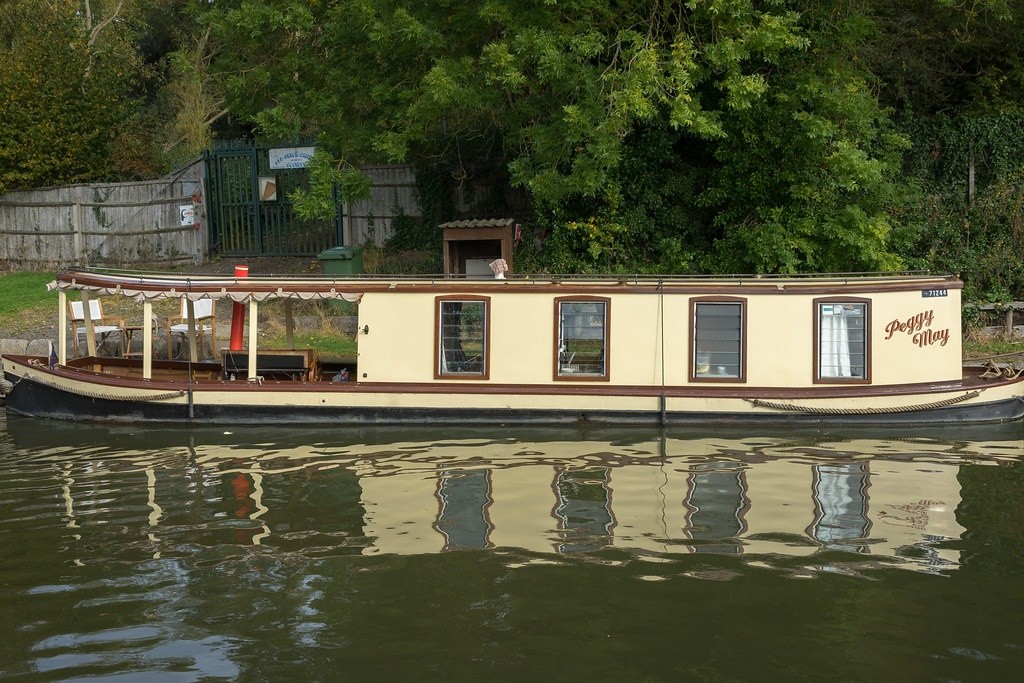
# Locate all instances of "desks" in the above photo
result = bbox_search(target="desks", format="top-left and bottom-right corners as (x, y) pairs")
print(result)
(116, 320), (159, 360)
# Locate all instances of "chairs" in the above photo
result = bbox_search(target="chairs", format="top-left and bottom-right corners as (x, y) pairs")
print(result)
(67, 298), (125, 358)
(165, 298), (216, 359)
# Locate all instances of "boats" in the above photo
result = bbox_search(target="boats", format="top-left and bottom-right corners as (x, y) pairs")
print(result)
(0, 265), (1024, 431)
